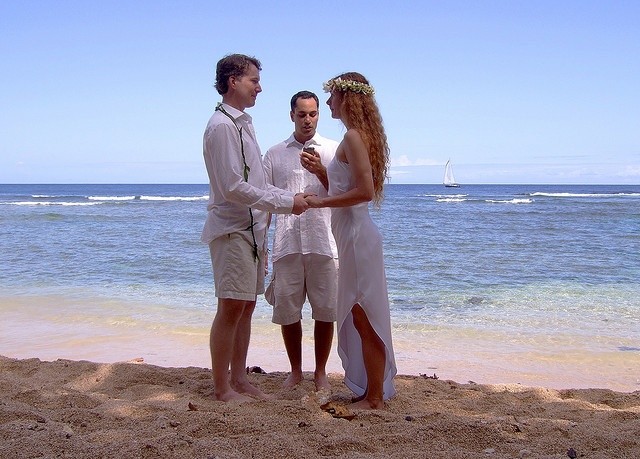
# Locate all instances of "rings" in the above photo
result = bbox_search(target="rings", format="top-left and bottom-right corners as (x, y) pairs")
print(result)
(305, 164), (308, 169)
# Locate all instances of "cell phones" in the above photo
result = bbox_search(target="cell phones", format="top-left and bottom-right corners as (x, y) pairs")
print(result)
(304, 147), (315, 156)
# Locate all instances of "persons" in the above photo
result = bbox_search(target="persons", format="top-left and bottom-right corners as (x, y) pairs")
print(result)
(203, 54), (317, 405)
(304, 72), (396, 418)
(262, 91), (339, 393)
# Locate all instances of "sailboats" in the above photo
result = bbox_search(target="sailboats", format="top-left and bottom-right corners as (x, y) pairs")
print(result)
(443, 157), (460, 187)
